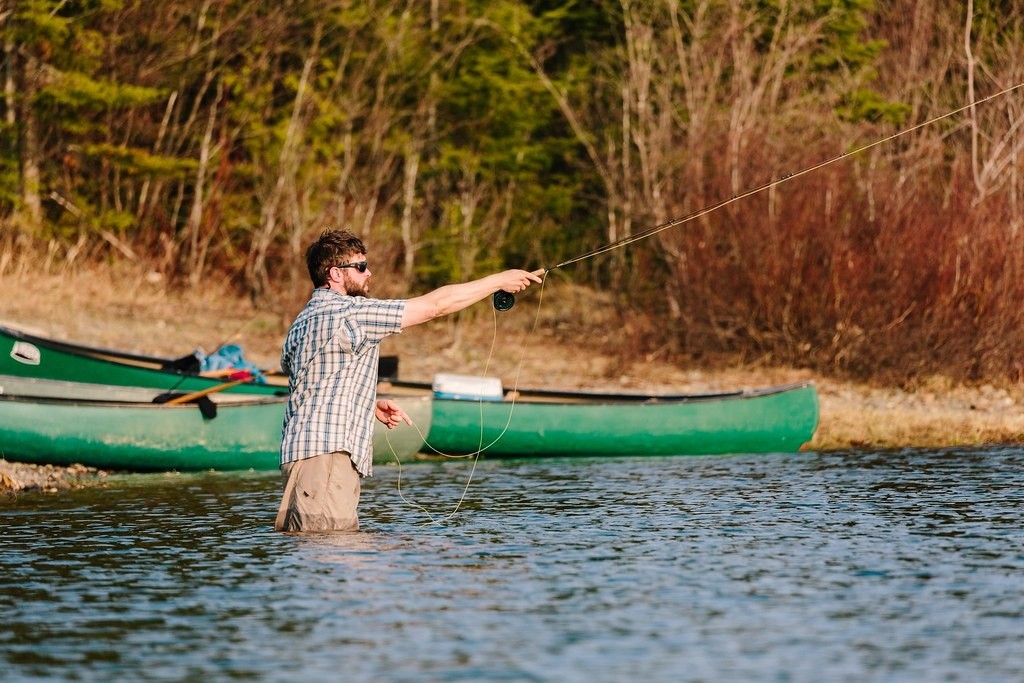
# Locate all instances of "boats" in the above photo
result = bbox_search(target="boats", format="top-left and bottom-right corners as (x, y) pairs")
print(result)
(13, 331), (820, 457)
(0, 328), (433, 470)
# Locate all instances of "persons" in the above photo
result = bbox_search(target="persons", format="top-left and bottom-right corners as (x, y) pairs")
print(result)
(274, 228), (542, 532)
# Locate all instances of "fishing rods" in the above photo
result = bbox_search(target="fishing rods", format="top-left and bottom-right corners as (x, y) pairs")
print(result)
(489, 84), (1023, 312)
(161, 368), (280, 404)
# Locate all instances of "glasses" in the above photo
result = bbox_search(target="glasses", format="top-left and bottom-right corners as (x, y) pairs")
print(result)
(325, 261), (367, 275)
